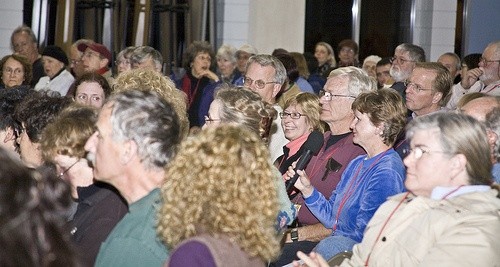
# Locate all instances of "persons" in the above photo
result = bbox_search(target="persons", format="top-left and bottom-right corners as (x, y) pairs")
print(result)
(0, 25), (500, 267)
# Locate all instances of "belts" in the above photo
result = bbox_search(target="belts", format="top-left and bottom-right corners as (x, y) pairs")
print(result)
(287, 221), (307, 229)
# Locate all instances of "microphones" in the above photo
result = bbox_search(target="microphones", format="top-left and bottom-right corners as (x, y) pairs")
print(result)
(286, 131), (324, 194)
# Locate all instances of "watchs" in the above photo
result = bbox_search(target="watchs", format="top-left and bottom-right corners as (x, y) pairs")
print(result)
(291, 228), (298, 242)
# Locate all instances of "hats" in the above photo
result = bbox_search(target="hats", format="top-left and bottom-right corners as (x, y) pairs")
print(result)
(38, 44), (69, 66)
(77, 43), (112, 61)
(338, 39), (359, 51)
(235, 44), (257, 56)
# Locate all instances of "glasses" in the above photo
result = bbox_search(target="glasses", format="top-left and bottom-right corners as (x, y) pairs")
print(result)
(81, 52), (99, 58)
(53, 158), (80, 180)
(237, 55), (249, 59)
(480, 57), (500, 66)
(15, 126), (28, 138)
(460, 65), (467, 70)
(389, 57), (416, 64)
(403, 146), (450, 160)
(404, 81), (434, 92)
(70, 59), (81, 64)
(204, 116), (221, 126)
(115, 60), (131, 66)
(243, 77), (279, 89)
(319, 89), (357, 102)
(198, 56), (212, 61)
(340, 48), (354, 53)
(280, 112), (306, 119)
